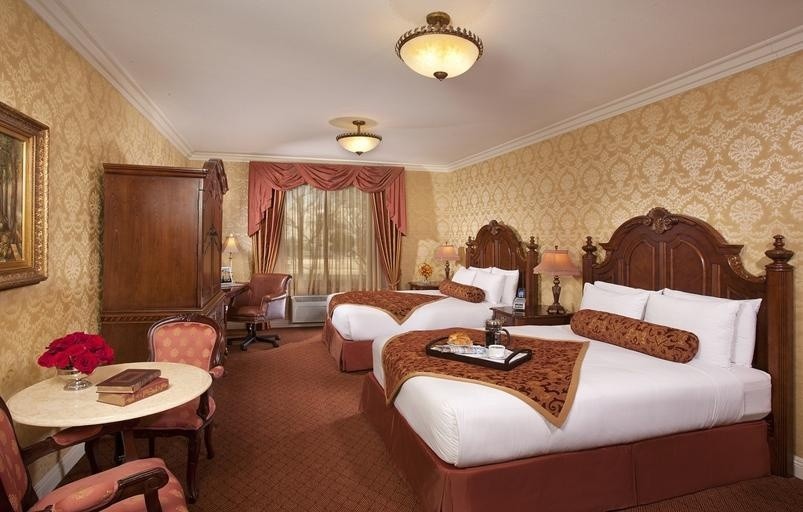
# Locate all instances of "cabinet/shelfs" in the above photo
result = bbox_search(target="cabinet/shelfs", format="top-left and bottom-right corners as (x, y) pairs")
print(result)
(101, 153), (227, 375)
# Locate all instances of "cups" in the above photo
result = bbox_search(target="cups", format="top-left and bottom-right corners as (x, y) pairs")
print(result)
(484, 318), (511, 349)
(487, 344), (506, 358)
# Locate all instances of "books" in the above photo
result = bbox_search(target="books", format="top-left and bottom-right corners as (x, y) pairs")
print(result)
(95, 368), (161, 395)
(96, 377), (170, 407)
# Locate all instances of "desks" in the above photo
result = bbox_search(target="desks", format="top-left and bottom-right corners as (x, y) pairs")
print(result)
(4, 360), (210, 464)
(221, 281), (248, 296)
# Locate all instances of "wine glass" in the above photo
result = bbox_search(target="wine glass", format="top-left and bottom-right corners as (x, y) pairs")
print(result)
(55, 366), (96, 392)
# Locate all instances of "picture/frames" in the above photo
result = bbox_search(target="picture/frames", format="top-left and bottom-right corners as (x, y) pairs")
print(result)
(0, 101), (52, 291)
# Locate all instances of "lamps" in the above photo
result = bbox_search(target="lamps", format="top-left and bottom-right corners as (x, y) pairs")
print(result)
(433, 239), (459, 281)
(222, 231), (240, 283)
(333, 118), (382, 157)
(391, 10), (485, 84)
(532, 239), (580, 317)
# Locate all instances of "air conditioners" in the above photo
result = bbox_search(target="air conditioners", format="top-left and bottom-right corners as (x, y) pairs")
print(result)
(289, 295), (327, 323)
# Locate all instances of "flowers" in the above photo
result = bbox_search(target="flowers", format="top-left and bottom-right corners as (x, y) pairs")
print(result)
(33, 329), (115, 377)
(416, 262), (432, 279)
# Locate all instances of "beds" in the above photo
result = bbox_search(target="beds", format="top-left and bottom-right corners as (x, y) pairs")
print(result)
(357, 207), (799, 510)
(320, 218), (538, 374)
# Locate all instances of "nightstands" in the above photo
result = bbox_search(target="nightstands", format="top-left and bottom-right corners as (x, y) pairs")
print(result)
(488, 302), (573, 328)
(407, 281), (440, 289)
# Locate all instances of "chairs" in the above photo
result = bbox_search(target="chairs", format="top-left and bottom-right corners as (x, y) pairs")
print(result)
(224, 272), (292, 351)
(0, 397), (187, 512)
(119, 317), (224, 505)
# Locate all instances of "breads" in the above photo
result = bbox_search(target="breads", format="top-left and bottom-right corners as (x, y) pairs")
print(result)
(447, 331), (471, 346)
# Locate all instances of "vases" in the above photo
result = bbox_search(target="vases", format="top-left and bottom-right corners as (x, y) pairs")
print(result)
(53, 364), (91, 391)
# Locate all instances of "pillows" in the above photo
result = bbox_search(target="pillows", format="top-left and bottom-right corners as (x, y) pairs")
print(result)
(568, 274), (767, 369)
(439, 258), (521, 305)
